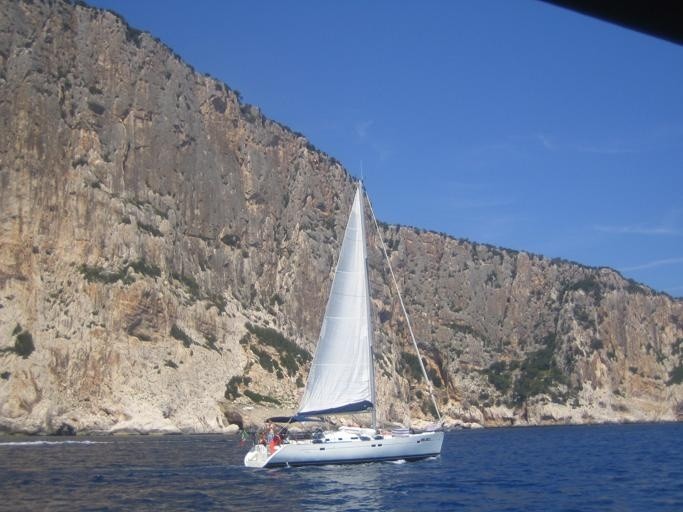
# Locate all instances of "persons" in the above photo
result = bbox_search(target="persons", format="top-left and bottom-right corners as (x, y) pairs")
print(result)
(267, 419), (274, 440)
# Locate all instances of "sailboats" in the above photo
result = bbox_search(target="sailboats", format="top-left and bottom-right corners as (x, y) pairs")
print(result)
(244, 172), (442, 471)
